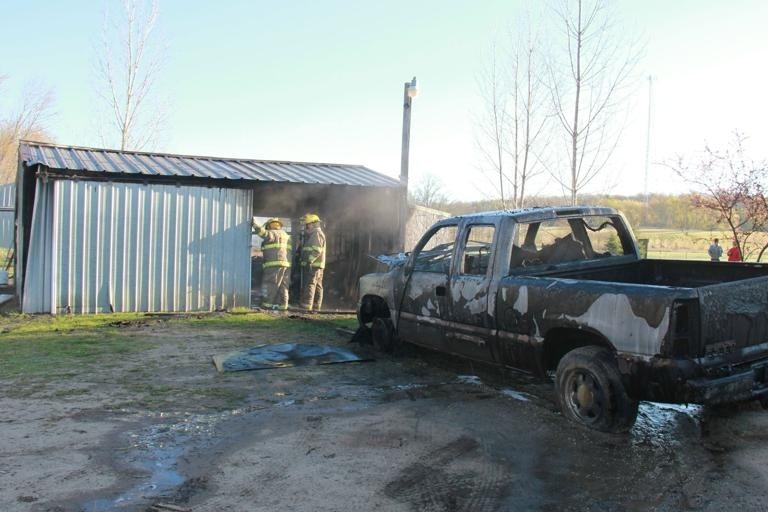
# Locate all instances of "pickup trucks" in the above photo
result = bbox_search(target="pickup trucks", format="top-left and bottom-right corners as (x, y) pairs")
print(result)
(355, 205), (768, 436)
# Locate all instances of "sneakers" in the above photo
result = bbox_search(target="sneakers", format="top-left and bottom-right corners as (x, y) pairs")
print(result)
(261, 303), (322, 315)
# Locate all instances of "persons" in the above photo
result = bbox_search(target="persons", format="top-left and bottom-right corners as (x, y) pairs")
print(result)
(297, 214), (326, 315)
(727, 241), (740, 262)
(708, 239), (723, 262)
(252, 216), (292, 315)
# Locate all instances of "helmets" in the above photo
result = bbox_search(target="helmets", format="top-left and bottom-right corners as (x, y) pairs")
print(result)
(265, 218), (283, 230)
(300, 214), (321, 226)
